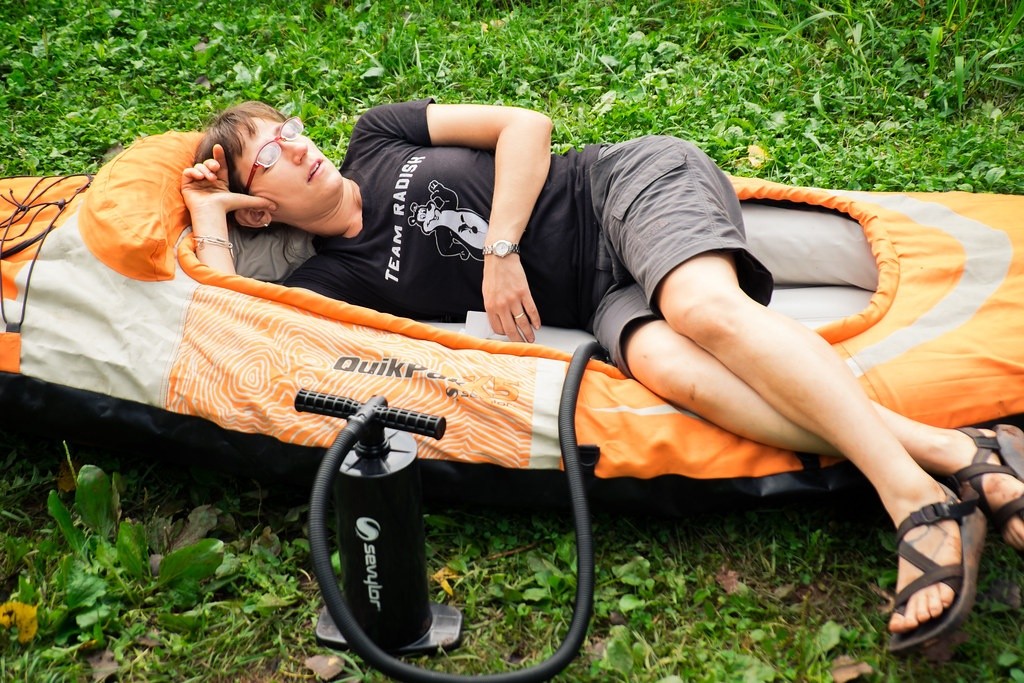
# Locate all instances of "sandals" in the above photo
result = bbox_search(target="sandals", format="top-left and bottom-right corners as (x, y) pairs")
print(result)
(947, 424), (1024, 562)
(888, 482), (985, 653)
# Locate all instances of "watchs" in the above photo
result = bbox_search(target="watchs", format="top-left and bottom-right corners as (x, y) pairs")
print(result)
(482, 240), (520, 258)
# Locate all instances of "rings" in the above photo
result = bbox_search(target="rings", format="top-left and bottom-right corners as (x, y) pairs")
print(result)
(514, 312), (524, 318)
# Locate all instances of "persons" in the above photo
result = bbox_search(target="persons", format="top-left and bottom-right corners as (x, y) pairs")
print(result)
(180, 99), (1024, 651)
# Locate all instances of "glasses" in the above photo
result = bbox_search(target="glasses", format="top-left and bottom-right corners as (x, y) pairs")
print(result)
(245, 116), (304, 195)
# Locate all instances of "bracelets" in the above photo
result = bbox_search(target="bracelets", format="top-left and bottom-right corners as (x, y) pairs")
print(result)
(193, 235), (233, 249)
